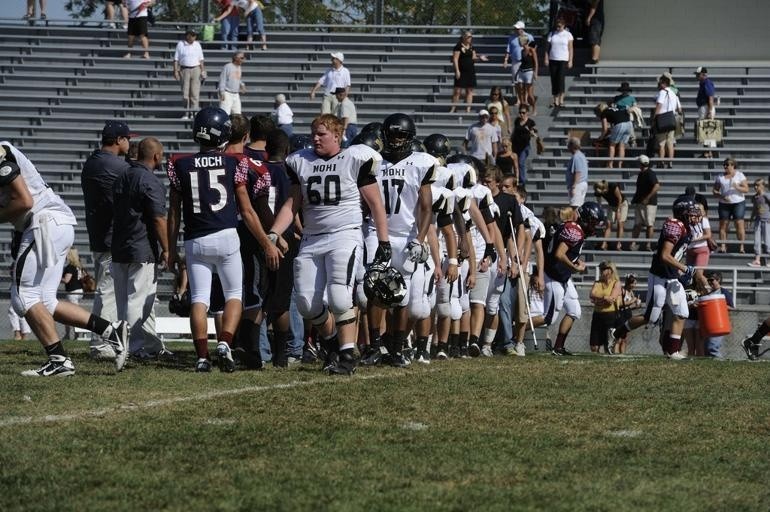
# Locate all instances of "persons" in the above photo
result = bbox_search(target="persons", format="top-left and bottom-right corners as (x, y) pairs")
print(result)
(0, 1), (769, 378)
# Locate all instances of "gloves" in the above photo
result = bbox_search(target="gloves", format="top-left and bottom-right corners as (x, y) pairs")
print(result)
(678, 272), (694, 289)
(372, 240), (393, 266)
(684, 265), (696, 277)
(403, 239), (423, 264)
(420, 241), (430, 263)
(265, 230), (280, 247)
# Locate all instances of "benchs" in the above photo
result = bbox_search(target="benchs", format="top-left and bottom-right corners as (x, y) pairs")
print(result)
(74, 317), (217, 343)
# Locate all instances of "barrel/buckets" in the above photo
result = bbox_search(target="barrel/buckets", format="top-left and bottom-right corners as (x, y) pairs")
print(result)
(698, 294), (732, 336)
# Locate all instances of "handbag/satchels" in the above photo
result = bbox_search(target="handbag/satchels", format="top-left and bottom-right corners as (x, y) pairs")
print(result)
(615, 305), (633, 325)
(81, 274), (96, 293)
(693, 118), (724, 143)
(653, 111), (677, 135)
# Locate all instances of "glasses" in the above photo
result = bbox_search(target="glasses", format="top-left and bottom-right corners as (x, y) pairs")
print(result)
(518, 111), (527, 115)
(489, 111), (498, 115)
(722, 163), (734, 166)
(706, 278), (718, 282)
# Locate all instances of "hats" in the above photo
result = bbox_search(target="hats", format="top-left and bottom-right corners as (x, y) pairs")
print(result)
(463, 32), (473, 41)
(101, 120), (142, 147)
(329, 51), (345, 62)
(615, 81), (633, 93)
(512, 20), (526, 30)
(656, 71), (675, 86)
(692, 66), (708, 74)
(185, 28), (197, 36)
(635, 154), (651, 166)
(329, 87), (346, 96)
(479, 108), (491, 117)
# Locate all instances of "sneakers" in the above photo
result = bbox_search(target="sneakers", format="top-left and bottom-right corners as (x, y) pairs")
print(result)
(320, 333), (339, 373)
(450, 345), (460, 359)
(481, 346), (494, 358)
(459, 346), (469, 359)
(304, 341), (320, 363)
(214, 341), (236, 374)
(436, 346), (448, 360)
(501, 348), (519, 357)
(514, 343), (526, 357)
(746, 260), (761, 268)
(195, 358), (212, 374)
(666, 351), (691, 361)
(101, 318), (131, 373)
(359, 345), (413, 368)
(469, 342), (481, 358)
(327, 349), (358, 377)
(606, 326), (618, 356)
(740, 335), (763, 363)
(138, 347), (175, 360)
(551, 348), (574, 357)
(19, 354), (77, 379)
(415, 349), (431, 365)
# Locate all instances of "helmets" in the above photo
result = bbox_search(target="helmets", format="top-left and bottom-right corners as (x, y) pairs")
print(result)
(349, 134), (383, 152)
(191, 105), (234, 151)
(671, 196), (701, 227)
(360, 120), (382, 135)
(379, 113), (418, 158)
(451, 144), (467, 154)
(446, 154), (485, 185)
(288, 133), (314, 154)
(363, 264), (409, 306)
(426, 133), (452, 157)
(576, 200), (604, 235)
(410, 139), (427, 153)
